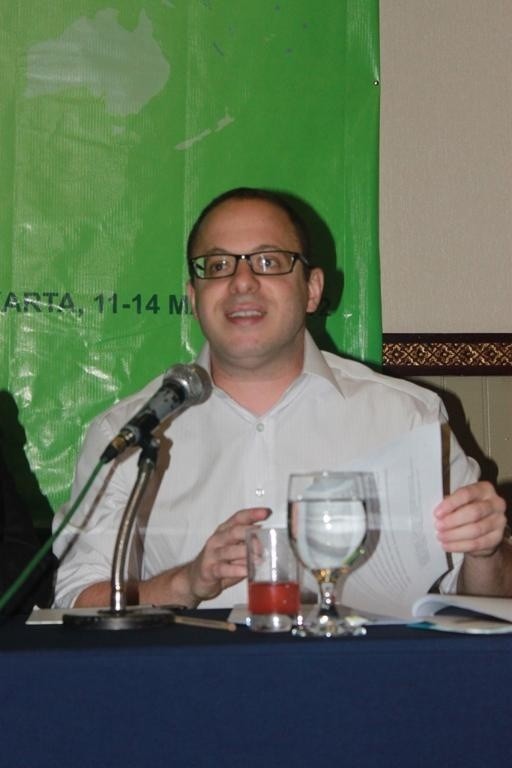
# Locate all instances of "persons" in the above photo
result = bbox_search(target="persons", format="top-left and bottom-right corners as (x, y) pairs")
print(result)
(1, 386), (58, 627)
(49, 184), (512, 619)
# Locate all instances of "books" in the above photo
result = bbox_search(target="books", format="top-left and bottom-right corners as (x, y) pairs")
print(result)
(174, 608), (252, 632)
(335, 593), (511, 636)
(26, 604), (173, 625)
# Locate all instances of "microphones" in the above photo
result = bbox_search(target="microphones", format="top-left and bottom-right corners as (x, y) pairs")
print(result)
(97, 362), (204, 462)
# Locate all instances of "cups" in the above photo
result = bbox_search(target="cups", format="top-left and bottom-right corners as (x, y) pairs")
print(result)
(246, 527), (303, 636)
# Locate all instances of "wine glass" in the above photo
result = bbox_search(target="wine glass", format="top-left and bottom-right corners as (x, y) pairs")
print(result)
(288, 472), (367, 639)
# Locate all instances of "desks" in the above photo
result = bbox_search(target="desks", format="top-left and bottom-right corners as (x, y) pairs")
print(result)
(0, 606), (512, 768)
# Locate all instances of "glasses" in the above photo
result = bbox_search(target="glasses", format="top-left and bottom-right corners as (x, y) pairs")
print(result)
(188, 249), (310, 279)
